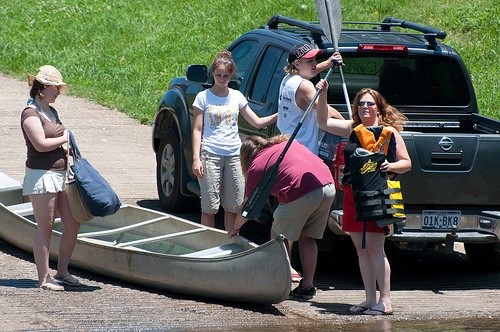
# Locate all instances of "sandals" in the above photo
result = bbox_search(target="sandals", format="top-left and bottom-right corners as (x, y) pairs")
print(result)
(54, 274), (82, 286)
(41, 278), (65, 291)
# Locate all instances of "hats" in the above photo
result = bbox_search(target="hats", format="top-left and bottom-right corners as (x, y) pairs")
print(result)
(28, 65), (68, 92)
(288, 44), (322, 65)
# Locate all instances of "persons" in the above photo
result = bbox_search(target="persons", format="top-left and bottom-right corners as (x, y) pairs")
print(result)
(316, 79), (412, 315)
(192, 51), (278, 234)
(229, 135), (336, 300)
(277, 43), (343, 285)
(21, 64), (80, 291)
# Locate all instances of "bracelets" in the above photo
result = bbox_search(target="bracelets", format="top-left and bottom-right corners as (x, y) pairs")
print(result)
(233, 228), (240, 231)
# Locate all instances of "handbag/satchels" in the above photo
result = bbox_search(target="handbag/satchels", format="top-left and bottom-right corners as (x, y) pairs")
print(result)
(69, 131), (122, 216)
(64, 131), (93, 222)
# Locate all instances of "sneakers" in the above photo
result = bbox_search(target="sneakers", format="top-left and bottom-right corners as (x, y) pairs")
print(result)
(292, 278), (316, 299)
(288, 292), (294, 300)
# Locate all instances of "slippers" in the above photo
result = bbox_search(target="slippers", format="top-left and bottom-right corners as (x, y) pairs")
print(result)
(347, 305), (366, 315)
(362, 307), (394, 315)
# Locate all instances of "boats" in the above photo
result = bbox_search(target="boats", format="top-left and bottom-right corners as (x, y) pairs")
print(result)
(0, 171), (292, 304)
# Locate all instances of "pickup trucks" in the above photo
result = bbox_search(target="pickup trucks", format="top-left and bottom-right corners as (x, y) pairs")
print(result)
(151, 14), (500, 256)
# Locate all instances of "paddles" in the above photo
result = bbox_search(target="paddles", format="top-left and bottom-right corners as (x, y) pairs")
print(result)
(241, 59), (340, 221)
(314, 0), (352, 120)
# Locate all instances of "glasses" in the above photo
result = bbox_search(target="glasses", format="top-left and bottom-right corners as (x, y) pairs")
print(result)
(358, 101), (375, 107)
